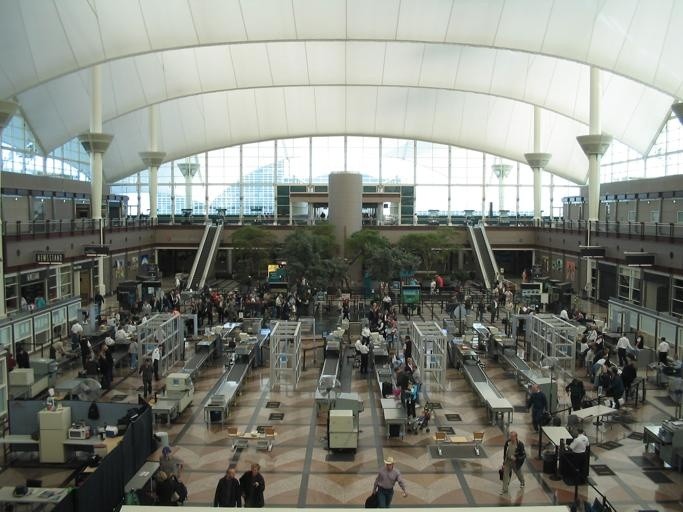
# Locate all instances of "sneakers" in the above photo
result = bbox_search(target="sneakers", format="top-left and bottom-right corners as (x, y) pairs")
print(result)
(500, 489), (508, 495)
(520, 480), (524, 488)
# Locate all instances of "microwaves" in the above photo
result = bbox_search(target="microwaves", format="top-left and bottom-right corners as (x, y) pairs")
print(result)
(68, 426), (90, 439)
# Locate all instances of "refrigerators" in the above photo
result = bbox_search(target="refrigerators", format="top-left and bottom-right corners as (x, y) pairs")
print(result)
(38, 406), (71, 463)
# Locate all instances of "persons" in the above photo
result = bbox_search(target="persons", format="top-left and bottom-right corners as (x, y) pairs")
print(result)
(33, 290), (45, 310)
(566, 377), (585, 411)
(497, 430), (527, 495)
(371, 455), (408, 508)
(50, 278), (241, 397)
(466, 268), (535, 323)
(655, 336), (670, 364)
(547, 306), (644, 400)
(568, 427), (590, 485)
(355, 312), (422, 418)
(213, 466), (241, 507)
(526, 384), (549, 432)
(238, 462), (265, 507)
(4, 348), (13, 371)
(247, 278), (395, 320)
(154, 470), (178, 506)
(15, 347), (29, 368)
(158, 446), (183, 481)
(430, 274), (443, 295)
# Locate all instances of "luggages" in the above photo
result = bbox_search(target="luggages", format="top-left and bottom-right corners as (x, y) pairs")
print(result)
(383, 381), (392, 398)
(581, 397), (592, 408)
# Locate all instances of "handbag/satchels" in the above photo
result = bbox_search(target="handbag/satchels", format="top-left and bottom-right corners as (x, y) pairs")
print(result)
(365, 492), (378, 508)
(543, 412), (551, 424)
(500, 469), (511, 480)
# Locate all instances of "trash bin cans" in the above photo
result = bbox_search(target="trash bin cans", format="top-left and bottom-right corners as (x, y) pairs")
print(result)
(155, 432), (168, 447)
(543, 450), (556, 473)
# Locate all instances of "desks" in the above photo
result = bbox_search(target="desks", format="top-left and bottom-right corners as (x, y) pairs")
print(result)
(571, 405), (618, 426)
(63, 435), (107, 456)
(228, 431), (277, 452)
(0, 435), (39, 466)
(535, 426), (572, 481)
(433, 436), (482, 456)
(0, 487), (68, 505)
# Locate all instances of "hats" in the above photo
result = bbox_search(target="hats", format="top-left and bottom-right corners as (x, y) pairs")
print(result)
(384, 457), (393, 464)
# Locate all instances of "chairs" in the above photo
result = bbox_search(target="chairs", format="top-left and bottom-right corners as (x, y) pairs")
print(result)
(26, 480), (42, 487)
